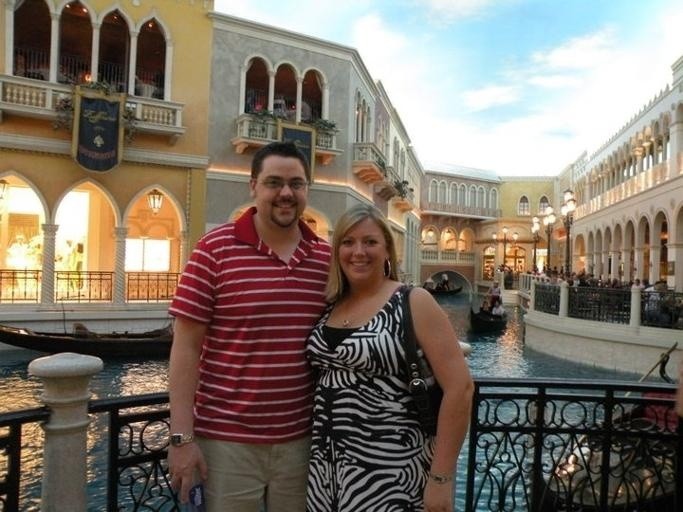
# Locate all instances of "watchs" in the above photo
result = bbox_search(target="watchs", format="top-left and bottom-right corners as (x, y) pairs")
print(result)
(166, 432), (194, 447)
(426, 472), (453, 483)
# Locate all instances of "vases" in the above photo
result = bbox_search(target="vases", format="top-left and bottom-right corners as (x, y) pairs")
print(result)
(136, 84), (157, 97)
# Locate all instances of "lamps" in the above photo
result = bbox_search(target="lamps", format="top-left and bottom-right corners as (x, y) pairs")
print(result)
(0, 178), (9, 203)
(147, 188), (164, 216)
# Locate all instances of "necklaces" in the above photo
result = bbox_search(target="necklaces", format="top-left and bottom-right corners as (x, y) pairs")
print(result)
(343, 317), (352, 327)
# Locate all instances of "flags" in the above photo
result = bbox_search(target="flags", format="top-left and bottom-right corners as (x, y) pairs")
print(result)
(71, 85), (128, 175)
(278, 121), (317, 186)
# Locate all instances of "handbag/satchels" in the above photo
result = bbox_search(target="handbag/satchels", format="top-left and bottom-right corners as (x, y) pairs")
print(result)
(408, 355), (445, 438)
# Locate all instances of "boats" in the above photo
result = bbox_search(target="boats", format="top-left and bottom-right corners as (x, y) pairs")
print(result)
(468, 307), (508, 331)
(424, 285), (464, 296)
(0, 318), (173, 363)
(521, 381), (682, 511)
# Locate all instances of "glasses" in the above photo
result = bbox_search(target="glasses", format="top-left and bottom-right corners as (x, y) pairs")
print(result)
(257, 176), (311, 192)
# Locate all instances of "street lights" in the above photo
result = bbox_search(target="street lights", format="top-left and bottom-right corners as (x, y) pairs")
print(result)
(541, 204), (557, 278)
(559, 187), (577, 276)
(512, 231), (521, 271)
(530, 216), (542, 274)
(501, 225), (509, 267)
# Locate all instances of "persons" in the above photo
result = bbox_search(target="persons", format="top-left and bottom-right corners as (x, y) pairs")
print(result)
(531, 263), (682, 329)
(299, 203), (476, 512)
(434, 282), (441, 291)
(440, 272), (449, 290)
(488, 281), (501, 304)
(480, 300), (490, 315)
(491, 300), (505, 318)
(161, 136), (346, 510)
